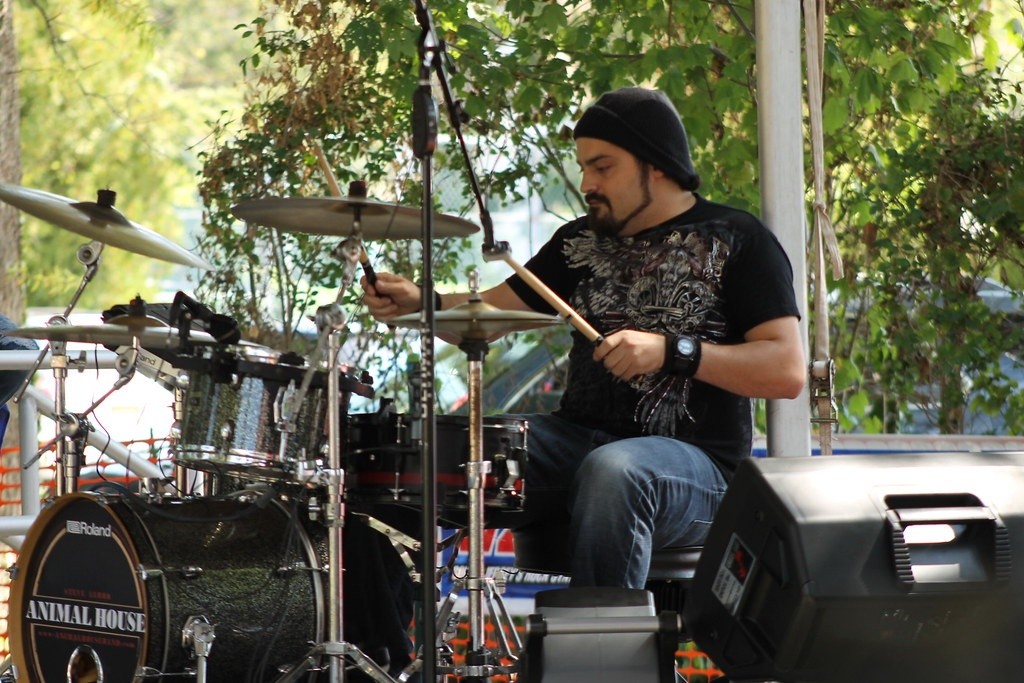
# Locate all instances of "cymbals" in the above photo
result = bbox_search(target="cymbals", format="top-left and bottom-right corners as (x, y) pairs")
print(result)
(3, 315), (221, 353)
(230, 181), (480, 243)
(380, 295), (565, 355)
(0, 184), (217, 276)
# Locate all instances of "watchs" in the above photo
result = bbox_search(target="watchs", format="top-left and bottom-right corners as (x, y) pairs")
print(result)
(660, 332), (701, 378)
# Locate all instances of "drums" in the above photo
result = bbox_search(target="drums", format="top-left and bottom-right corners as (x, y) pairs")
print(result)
(6, 488), (354, 683)
(338, 409), (531, 519)
(167, 344), (372, 491)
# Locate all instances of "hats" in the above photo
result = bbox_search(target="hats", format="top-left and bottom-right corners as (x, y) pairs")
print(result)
(573, 87), (700, 191)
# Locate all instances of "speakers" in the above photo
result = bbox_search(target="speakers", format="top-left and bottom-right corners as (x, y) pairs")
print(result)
(685, 454), (1024, 683)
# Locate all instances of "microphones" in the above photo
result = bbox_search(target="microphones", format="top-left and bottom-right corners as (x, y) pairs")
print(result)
(178, 291), (241, 347)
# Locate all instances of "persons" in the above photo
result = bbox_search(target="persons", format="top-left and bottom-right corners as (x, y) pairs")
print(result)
(358, 86), (809, 590)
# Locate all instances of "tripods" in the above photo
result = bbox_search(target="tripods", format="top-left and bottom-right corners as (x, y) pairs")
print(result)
(399, 335), (525, 683)
(276, 218), (396, 683)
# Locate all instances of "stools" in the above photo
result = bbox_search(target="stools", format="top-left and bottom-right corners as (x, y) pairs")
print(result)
(511, 528), (706, 611)
